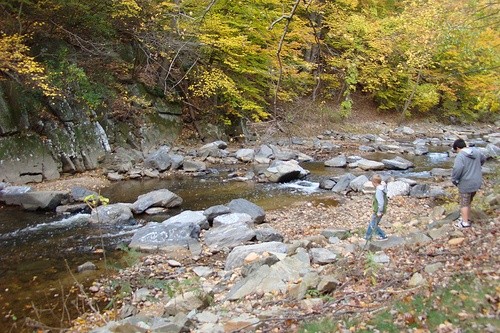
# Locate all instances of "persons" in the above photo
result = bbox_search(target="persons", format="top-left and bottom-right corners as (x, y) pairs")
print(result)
(365, 175), (389, 241)
(450, 139), (486, 229)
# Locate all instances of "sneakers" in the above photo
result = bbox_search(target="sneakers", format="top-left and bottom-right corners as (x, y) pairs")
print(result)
(376, 236), (388, 242)
(362, 238), (371, 242)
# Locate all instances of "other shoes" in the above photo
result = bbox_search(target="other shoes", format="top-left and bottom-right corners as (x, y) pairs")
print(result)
(455, 220), (470, 228)
